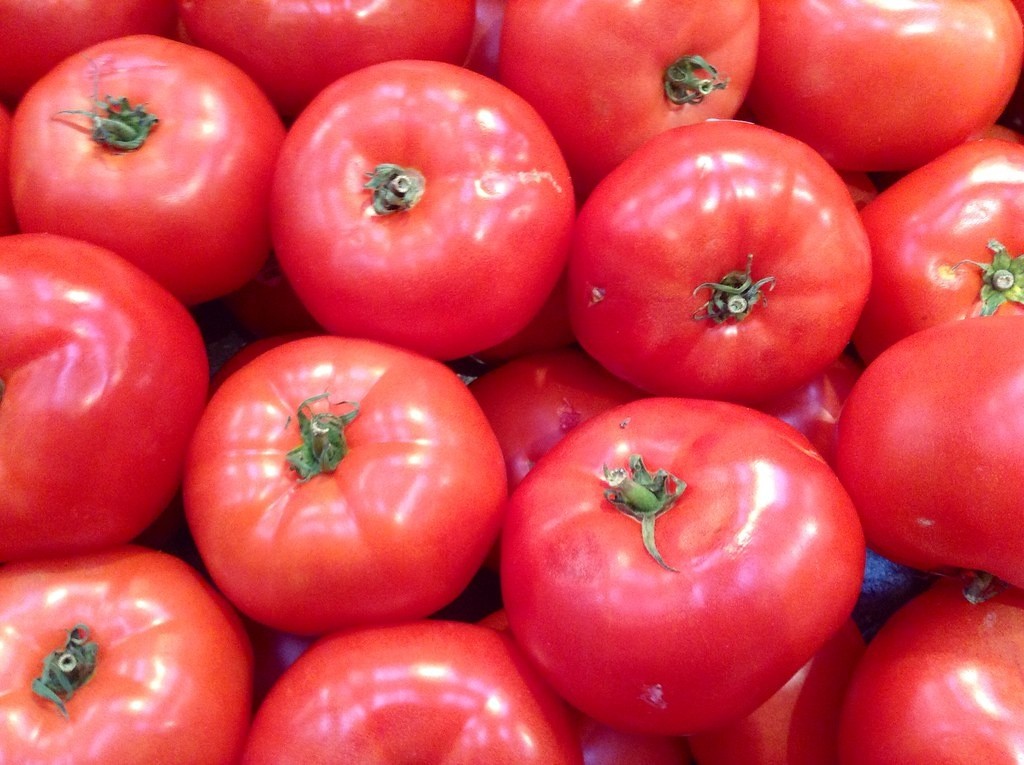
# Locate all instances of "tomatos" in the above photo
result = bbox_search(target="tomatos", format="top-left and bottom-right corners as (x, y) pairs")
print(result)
(0, 0), (1024, 765)
